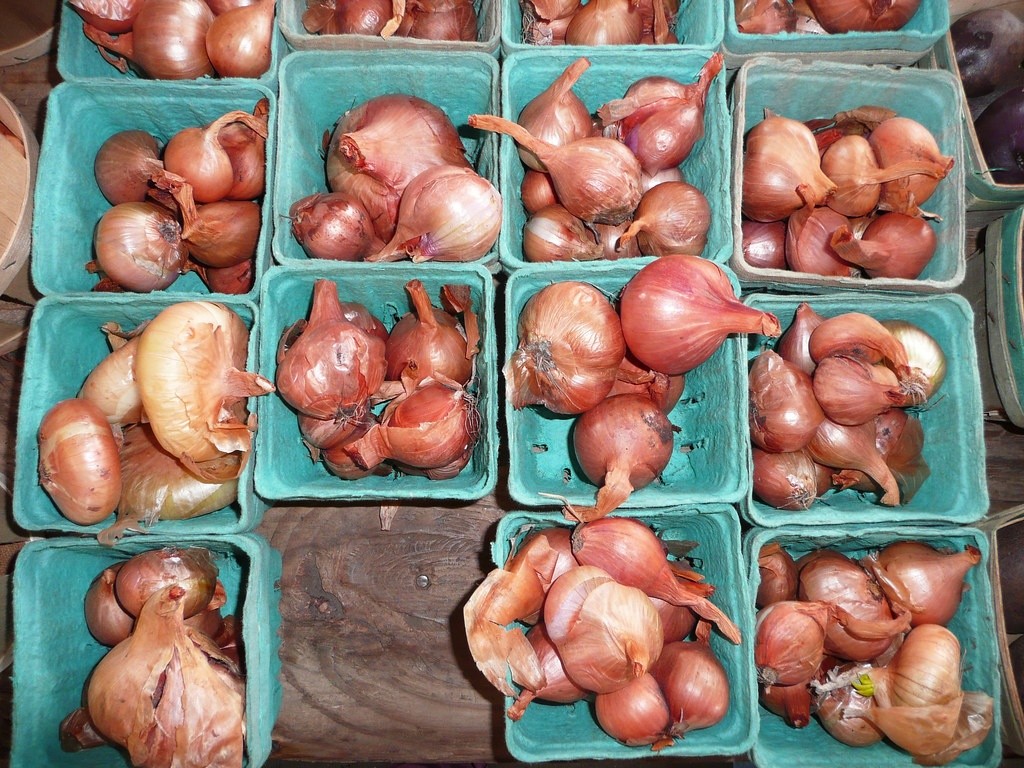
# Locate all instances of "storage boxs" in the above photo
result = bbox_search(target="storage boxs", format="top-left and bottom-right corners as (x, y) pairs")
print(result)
(739, 525), (1004, 768)
(722, 0), (950, 69)
(503, 259), (749, 511)
(8, 533), (282, 767)
(256, 266), (500, 504)
(272, 53), (504, 274)
(490, 505), (761, 763)
(501, 0), (725, 54)
(739, 292), (991, 532)
(277, 0), (504, 62)
(500, 49), (735, 265)
(31, 81), (276, 302)
(54, 0), (278, 91)
(12, 296), (268, 538)
(728, 59), (969, 293)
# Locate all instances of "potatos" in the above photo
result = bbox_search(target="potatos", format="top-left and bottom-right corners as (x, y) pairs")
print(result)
(948, 6), (1024, 186)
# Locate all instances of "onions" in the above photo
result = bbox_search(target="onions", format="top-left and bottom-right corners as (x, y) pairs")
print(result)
(27, 0), (980, 768)
